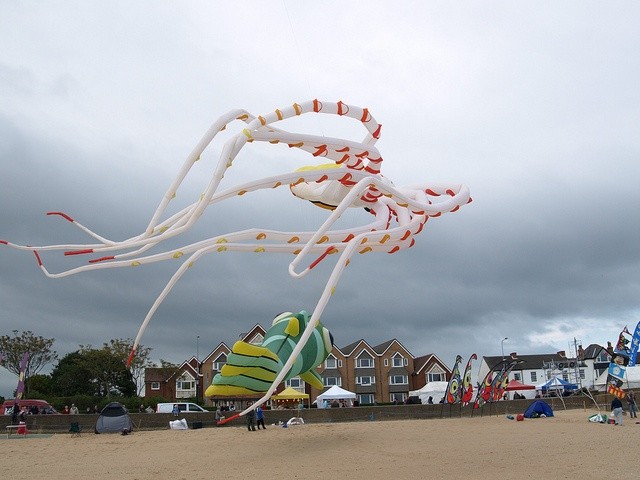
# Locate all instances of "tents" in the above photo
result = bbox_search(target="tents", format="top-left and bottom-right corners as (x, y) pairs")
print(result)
(95, 401), (132, 434)
(523, 400), (555, 418)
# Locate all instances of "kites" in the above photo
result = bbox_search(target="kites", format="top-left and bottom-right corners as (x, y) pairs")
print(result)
(14, 352), (29, 403)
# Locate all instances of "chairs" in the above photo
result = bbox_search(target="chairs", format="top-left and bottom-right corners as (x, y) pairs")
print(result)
(69, 421), (82, 437)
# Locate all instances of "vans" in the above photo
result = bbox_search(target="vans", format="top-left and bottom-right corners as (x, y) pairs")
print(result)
(156, 402), (209, 413)
(0, 399), (64, 415)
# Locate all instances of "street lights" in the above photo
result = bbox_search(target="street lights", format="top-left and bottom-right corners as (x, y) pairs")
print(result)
(195, 377), (200, 404)
(501, 338), (508, 368)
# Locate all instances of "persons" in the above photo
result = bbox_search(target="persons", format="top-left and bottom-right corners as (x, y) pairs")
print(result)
(146, 405), (155, 413)
(47, 405), (53, 414)
(552, 392), (556, 396)
(520, 393), (525, 399)
(357, 395), (360, 406)
(216, 406), (223, 427)
(62, 405), (70, 414)
(394, 400), (396, 405)
(172, 404), (180, 417)
(514, 391), (520, 399)
(427, 395), (434, 404)
(515, 374), (520, 380)
(93, 405), (101, 414)
(534, 392), (540, 398)
(28, 406), (39, 415)
(246, 402), (256, 431)
(255, 404), (267, 430)
(342, 403), (345, 407)
(353, 399), (355, 406)
(262, 404), (267, 410)
(285, 403), (290, 409)
(13, 404), (21, 415)
(625, 389), (638, 420)
(504, 394), (508, 400)
(225, 406), (229, 411)
(138, 404), (146, 413)
(70, 403), (79, 415)
(344, 401), (348, 407)
(228, 401), (236, 411)
(297, 402), (303, 418)
(540, 412), (547, 418)
(439, 396), (446, 403)
(42, 408), (46, 414)
(85, 407), (92, 414)
(221, 406), (223, 411)
(332, 399), (339, 407)
(563, 390), (570, 396)
(610, 395), (624, 427)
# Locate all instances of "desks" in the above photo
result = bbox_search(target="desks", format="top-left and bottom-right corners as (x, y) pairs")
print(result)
(6, 425), (27, 438)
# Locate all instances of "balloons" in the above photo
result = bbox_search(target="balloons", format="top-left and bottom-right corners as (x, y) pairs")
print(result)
(0, 91), (477, 424)
(203, 306), (337, 401)
